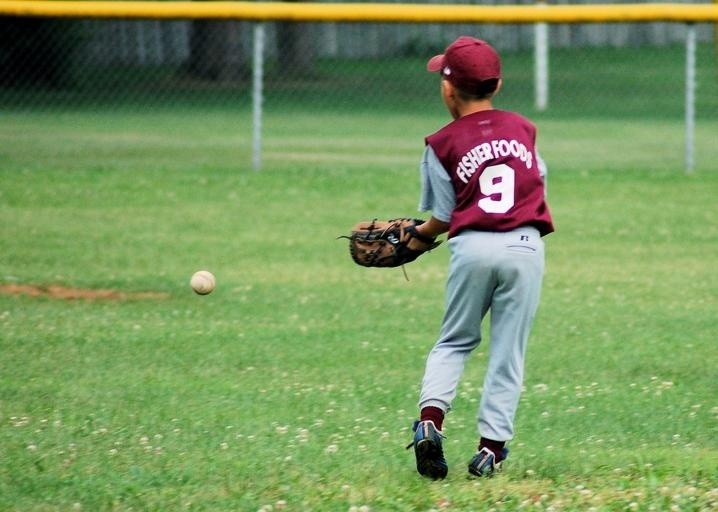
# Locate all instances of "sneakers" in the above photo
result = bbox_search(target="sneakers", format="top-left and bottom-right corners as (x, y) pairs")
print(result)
(468, 441), (510, 479)
(413, 417), (449, 482)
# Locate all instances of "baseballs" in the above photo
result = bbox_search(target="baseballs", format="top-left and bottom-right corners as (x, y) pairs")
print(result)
(190, 271), (215, 294)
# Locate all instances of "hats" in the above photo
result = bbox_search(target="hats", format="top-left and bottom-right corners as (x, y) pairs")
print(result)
(427, 36), (503, 98)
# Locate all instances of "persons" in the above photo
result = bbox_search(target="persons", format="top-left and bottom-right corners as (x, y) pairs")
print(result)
(336, 34), (555, 480)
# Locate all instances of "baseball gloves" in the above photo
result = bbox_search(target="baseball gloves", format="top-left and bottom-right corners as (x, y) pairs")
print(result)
(336, 217), (443, 280)
